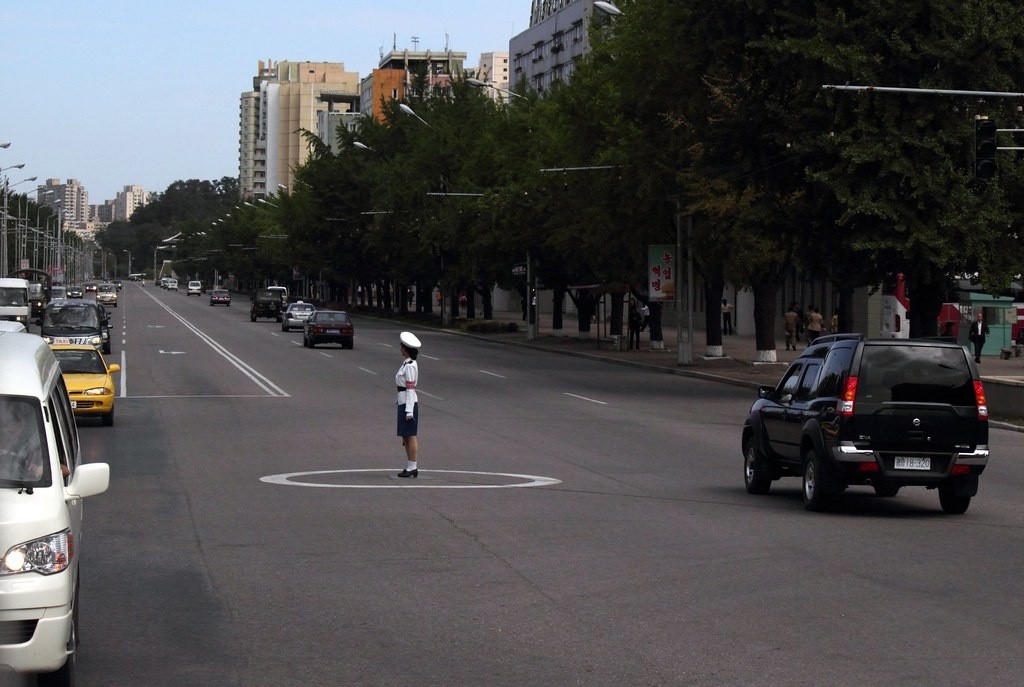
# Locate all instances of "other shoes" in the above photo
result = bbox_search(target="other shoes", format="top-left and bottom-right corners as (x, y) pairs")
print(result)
(786, 346), (790, 351)
(792, 346), (797, 351)
(974, 358), (981, 364)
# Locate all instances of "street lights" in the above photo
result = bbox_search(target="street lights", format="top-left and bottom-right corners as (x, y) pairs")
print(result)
(0, 163), (131, 287)
(211, 78), (535, 340)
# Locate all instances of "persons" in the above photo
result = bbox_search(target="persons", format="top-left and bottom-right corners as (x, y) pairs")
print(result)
(942, 322), (954, 342)
(629, 305), (650, 350)
(721, 298), (734, 336)
(784, 302), (838, 351)
(0, 401), (70, 482)
(437, 289), (442, 306)
(969, 312), (990, 363)
(396, 332), (421, 477)
(408, 287), (414, 307)
(459, 293), (467, 310)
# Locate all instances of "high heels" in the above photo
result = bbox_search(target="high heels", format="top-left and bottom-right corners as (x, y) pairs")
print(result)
(398, 469), (418, 478)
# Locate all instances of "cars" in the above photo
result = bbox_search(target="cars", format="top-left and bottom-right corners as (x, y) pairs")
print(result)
(49, 344), (120, 428)
(155, 278), (178, 291)
(209, 290), (231, 307)
(98, 303), (113, 354)
(302, 310), (354, 350)
(282, 303), (315, 332)
(0, 321), (27, 332)
(67, 280), (122, 307)
(187, 281), (201, 297)
(30, 282), (46, 318)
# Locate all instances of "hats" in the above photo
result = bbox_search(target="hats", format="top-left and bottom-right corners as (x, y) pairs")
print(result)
(400, 331), (421, 348)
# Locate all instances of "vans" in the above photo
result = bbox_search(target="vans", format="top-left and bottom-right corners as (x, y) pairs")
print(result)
(267, 287), (288, 312)
(41, 299), (110, 360)
(51, 287), (67, 314)
(0, 330), (109, 687)
(0, 278), (32, 333)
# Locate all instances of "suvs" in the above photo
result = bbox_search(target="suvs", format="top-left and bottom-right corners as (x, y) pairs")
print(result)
(741, 332), (989, 515)
(250, 289), (283, 321)
